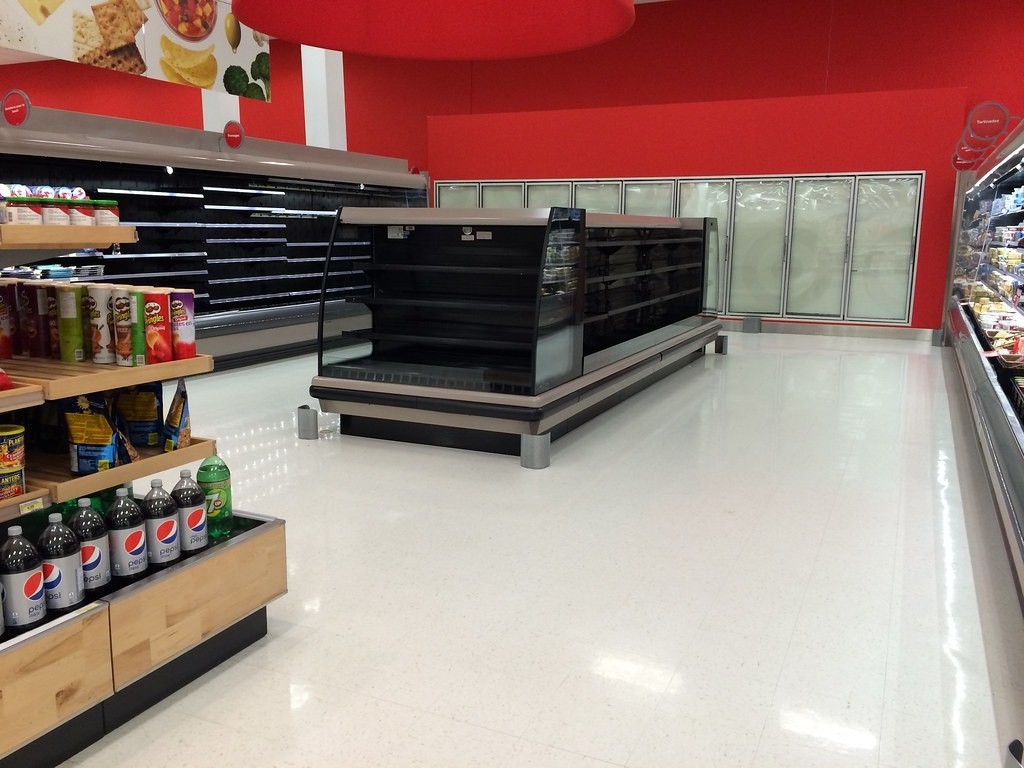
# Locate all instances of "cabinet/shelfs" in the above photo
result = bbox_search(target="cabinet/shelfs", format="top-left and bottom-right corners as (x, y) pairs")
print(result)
(0, 224), (288, 768)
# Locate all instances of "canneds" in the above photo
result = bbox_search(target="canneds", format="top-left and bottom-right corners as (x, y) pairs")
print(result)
(0, 424), (25, 501)
(0, 196), (120, 226)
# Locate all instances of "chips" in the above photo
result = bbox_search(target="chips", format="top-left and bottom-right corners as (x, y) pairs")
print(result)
(159, 35), (217, 89)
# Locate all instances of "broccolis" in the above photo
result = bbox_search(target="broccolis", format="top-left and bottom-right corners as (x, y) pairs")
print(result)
(224, 52), (271, 104)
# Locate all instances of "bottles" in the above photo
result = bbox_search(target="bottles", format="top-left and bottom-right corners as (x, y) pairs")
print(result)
(103, 487), (150, 589)
(36, 513), (86, 621)
(197, 448), (233, 542)
(68, 498), (113, 602)
(170, 470), (209, 558)
(140, 479), (181, 573)
(0, 526), (48, 645)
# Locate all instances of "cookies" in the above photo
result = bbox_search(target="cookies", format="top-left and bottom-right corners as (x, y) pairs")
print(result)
(70, 0), (149, 75)
